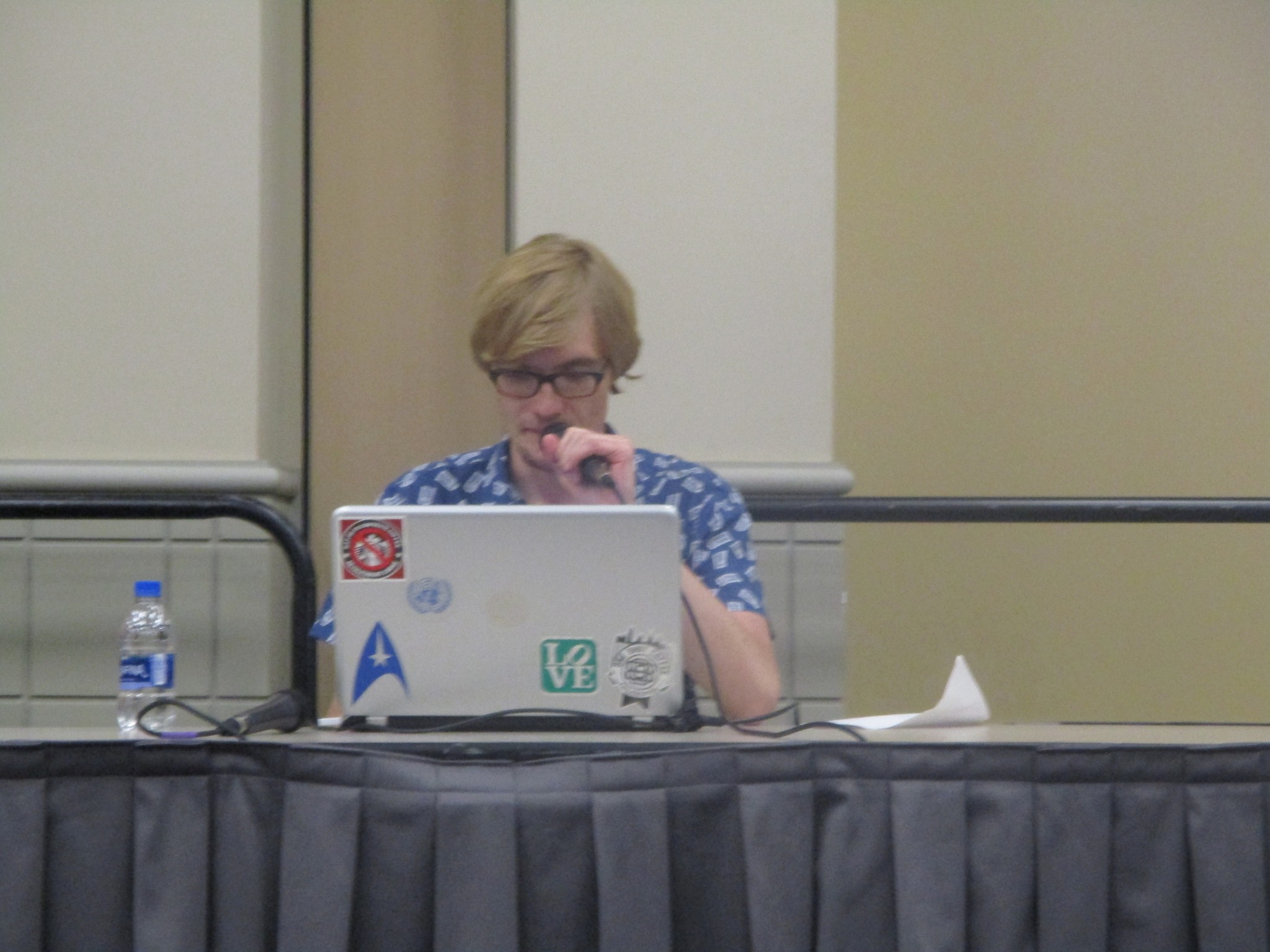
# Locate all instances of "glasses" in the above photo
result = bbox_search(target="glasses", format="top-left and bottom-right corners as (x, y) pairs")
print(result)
(482, 359), (611, 400)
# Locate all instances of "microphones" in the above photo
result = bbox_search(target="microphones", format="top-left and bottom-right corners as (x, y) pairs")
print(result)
(218, 687), (315, 733)
(541, 422), (611, 485)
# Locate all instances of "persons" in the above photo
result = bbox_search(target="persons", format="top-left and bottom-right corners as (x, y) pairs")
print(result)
(304, 230), (781, 724)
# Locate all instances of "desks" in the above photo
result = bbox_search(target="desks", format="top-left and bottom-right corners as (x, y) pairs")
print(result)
(0, 730), (1270, 952)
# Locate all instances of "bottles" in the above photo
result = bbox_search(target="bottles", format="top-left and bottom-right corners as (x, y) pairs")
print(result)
(116, 581), (177, 741)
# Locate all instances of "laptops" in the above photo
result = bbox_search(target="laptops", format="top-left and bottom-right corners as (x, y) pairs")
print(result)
(332, 503), (685, 726)
